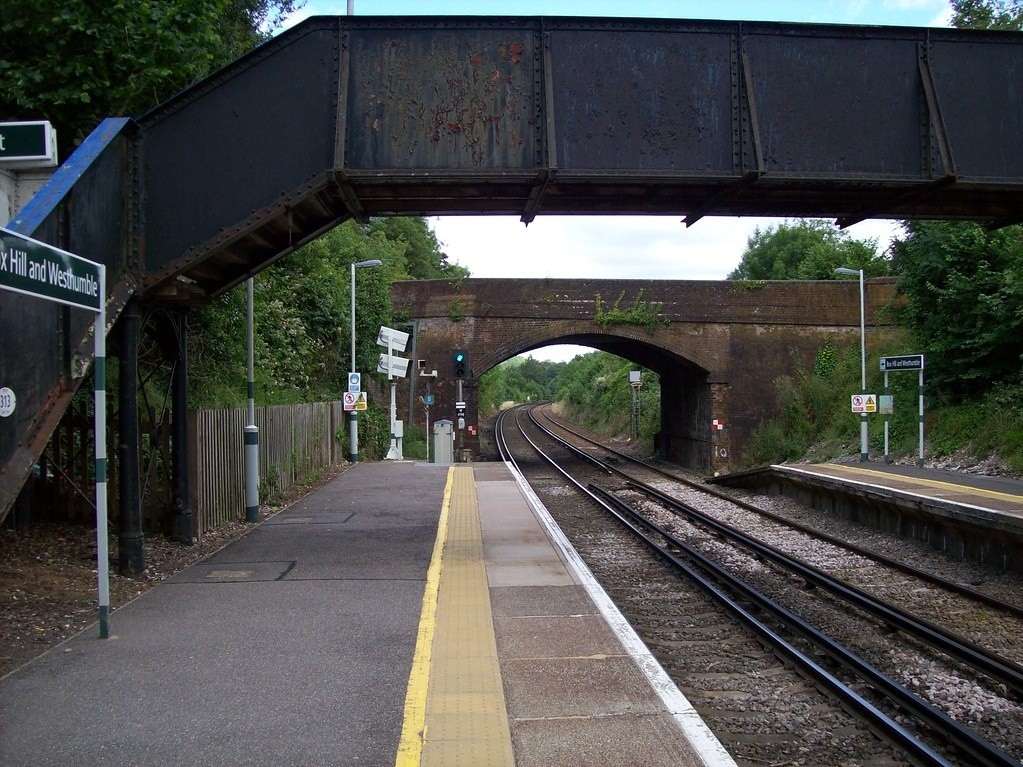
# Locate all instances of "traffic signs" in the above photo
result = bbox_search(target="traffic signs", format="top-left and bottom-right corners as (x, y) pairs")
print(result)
(878, 354), (924, 371)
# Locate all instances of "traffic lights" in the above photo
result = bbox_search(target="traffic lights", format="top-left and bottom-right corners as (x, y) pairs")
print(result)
(451, 349), (469, 378)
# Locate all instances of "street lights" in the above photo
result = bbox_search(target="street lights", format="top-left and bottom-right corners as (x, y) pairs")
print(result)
(836, 266), (872, 462)
(349, 259), (384, 465)
